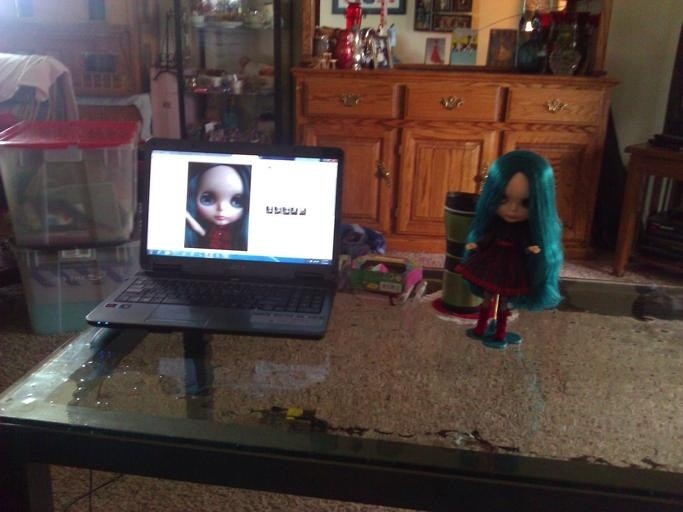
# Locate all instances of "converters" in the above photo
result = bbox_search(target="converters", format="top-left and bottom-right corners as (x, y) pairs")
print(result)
(266, 406), (317, 430)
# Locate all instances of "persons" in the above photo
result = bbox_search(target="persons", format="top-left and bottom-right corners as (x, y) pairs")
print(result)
(452, 149), (566, 350)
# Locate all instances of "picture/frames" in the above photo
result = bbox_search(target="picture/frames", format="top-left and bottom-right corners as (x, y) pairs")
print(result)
(331, 0), (473, 33)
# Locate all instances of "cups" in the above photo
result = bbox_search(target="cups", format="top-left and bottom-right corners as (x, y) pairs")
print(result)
(191, 15), (208, 28)
(231, 80), (244, 95)
(442, 191), (488, 313)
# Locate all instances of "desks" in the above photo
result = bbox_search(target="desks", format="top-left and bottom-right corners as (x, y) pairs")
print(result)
(0, 264), (683, 512)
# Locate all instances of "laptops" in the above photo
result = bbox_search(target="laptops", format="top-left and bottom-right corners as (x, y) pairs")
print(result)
(84, 136), (344, 340)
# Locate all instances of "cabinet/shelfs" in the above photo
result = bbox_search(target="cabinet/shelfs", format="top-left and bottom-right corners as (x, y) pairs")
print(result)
(291, 62), (620, 259)
(173, 0), (293, 147)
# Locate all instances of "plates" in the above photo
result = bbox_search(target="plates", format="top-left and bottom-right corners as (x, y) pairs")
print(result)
(208, 20), (243, 28)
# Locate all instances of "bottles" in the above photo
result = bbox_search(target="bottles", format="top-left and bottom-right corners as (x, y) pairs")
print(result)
(314, 28), (353, 69)
(346, 0), (362, 35)
(517, 18), (547, 74)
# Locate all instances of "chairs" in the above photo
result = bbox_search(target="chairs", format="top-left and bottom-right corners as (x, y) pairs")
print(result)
(612, 20), (683, 278)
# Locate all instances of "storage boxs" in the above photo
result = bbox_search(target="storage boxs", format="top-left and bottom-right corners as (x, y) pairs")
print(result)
(1, 24), (143, 126)
(1, 120), (139, 247)
(12, 241), (139, 336)
